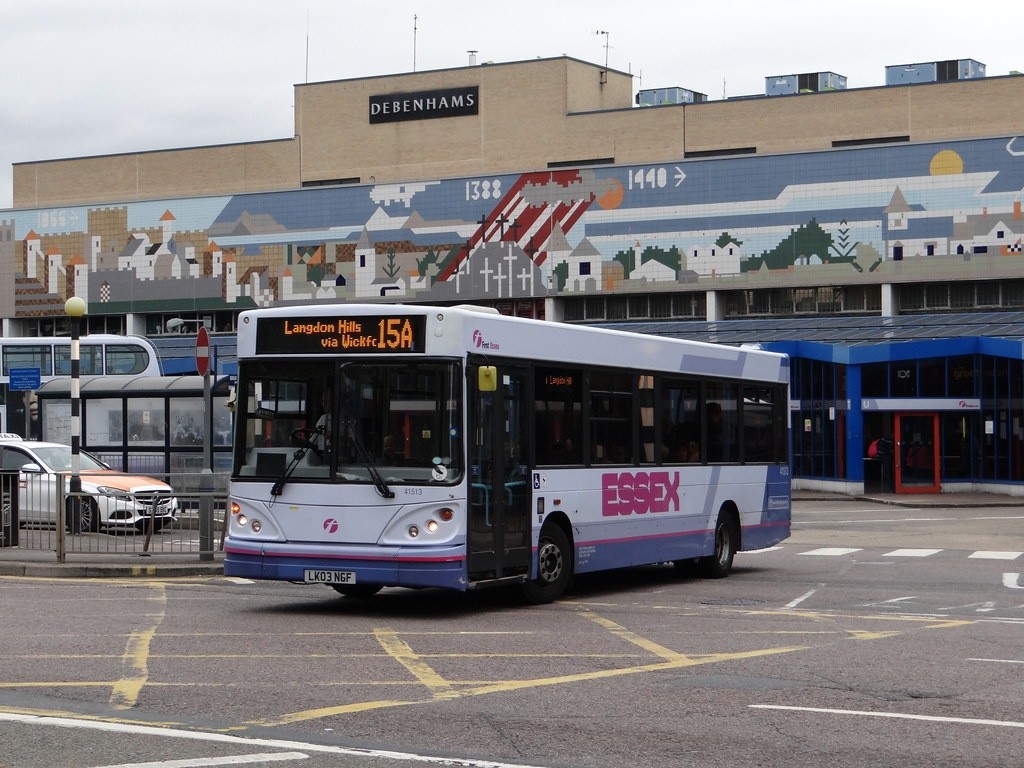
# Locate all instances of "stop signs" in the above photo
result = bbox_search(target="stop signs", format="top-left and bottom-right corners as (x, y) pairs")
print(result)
(195, 326), (210, 377)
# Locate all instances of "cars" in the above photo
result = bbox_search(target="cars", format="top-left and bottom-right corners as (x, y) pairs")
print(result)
(0, 432), (179, 535)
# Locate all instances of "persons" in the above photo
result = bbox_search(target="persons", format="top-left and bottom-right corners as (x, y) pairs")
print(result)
(308, 387), (356, 450)
(707, 401), (733, 447)
(384, 435), (399, 466)
(674, 441), (687, 461)
(879, 428), (895, 493)
(908, 441), (927, 478)
(21, 391), (38, 421)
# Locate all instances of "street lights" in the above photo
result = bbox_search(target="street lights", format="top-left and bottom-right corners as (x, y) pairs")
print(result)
(64, 296), (87, 531)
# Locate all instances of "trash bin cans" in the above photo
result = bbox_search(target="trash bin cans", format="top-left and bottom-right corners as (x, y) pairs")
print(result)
(0, 468), (19, 546)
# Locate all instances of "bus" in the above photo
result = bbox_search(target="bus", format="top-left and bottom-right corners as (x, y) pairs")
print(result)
(221, 303), (794, 607)
(0, 332), (166, 470)
